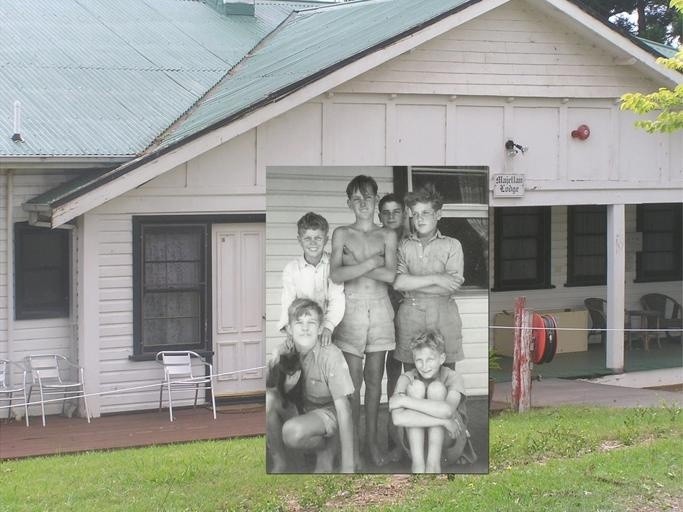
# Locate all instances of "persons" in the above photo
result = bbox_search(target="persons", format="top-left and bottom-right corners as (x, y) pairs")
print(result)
(391, 182), (467, 466)
(266, 299), (356, 474)
(330, 176), (398, 469)
(388, 329), (469, 473)
(358, 194), (409, 462)
(276, 211), (347, 347)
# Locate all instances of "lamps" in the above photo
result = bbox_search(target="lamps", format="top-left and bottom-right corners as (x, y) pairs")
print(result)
(505, 140), (528, 158)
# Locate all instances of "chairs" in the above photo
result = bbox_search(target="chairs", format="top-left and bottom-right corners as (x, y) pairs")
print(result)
(585, 293), (683, 352)
(0, 354), (91, 428)
(155, 350), (217, 423)
